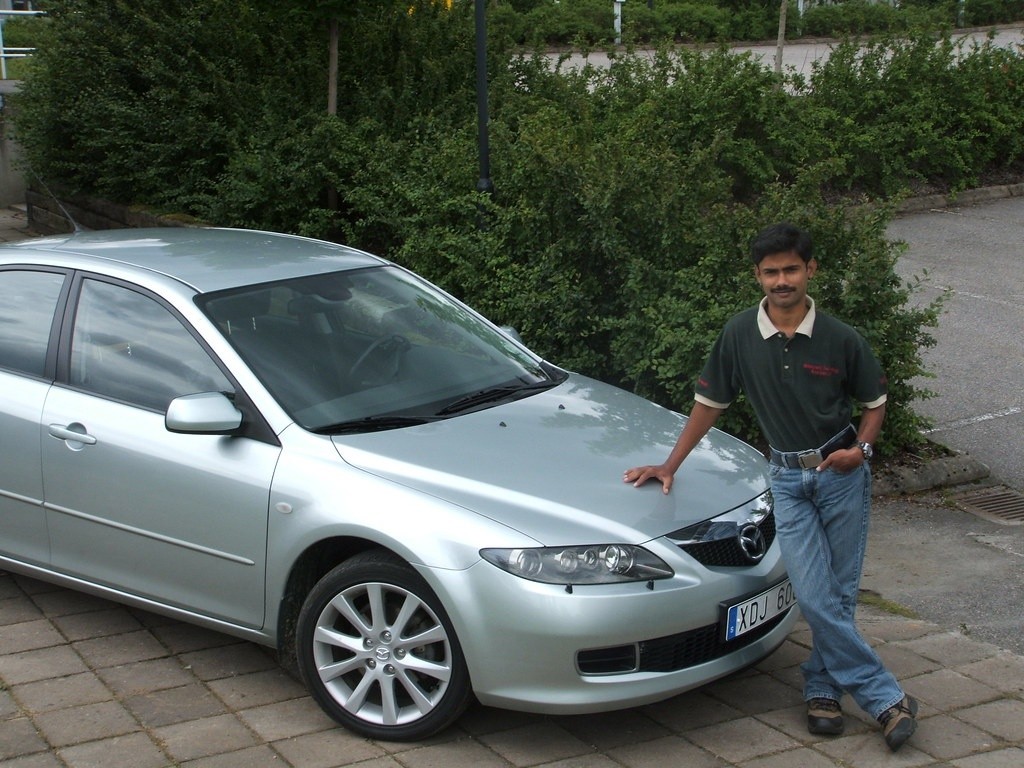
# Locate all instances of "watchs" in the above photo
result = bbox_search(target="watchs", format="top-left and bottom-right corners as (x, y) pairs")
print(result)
(853, 440), (873, 459)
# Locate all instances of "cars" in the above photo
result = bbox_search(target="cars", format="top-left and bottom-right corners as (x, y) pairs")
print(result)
(0, 226), (800, 742)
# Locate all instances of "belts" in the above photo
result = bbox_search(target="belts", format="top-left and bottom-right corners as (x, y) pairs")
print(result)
(770, 448), (823, 471)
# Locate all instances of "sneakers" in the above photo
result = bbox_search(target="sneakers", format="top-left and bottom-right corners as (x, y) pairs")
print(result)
(807, 697), (844, 735)
(877, 692), (918, 753)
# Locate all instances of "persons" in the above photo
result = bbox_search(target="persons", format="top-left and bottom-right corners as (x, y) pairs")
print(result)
(623, 225), (917, 752)
